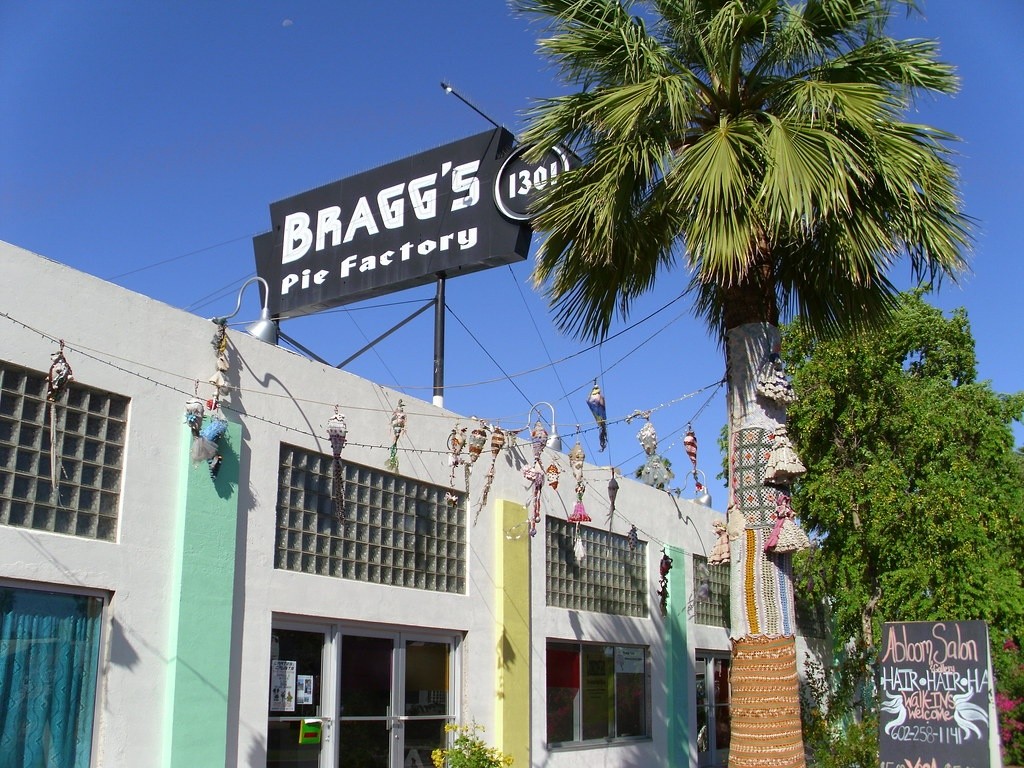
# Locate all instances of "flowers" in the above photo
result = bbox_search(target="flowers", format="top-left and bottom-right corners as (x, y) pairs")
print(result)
(431, 722), (514, 768)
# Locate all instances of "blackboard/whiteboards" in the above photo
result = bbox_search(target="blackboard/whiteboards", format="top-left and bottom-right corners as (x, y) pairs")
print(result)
(879, 621), (991, 768)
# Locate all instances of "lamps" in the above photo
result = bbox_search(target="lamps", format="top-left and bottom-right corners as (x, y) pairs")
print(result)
(674, 469), (712, 508)
(211, 277), (279, 346)
(506, 402), (562, 452)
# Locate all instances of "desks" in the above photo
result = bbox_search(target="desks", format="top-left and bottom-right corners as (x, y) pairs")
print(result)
(405, 738), (434, 768)
